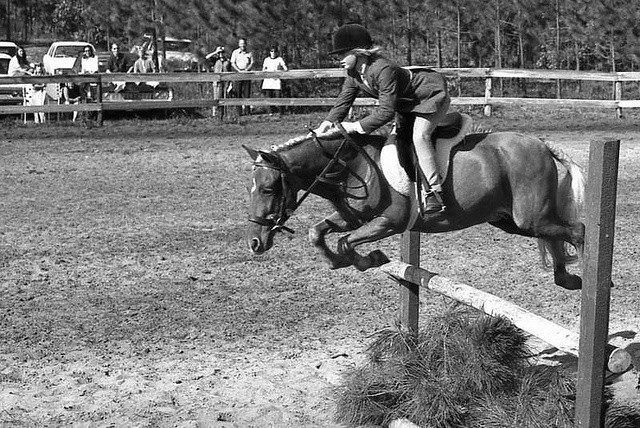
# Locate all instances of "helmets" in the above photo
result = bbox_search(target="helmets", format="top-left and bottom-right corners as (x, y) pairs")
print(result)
(327, 24), (372, 55)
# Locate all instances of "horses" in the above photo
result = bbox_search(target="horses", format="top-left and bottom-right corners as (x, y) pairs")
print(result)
(242, 130), (614, 290)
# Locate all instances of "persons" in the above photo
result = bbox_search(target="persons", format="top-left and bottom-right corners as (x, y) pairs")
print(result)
(105, 43), (127, 73)
(261, 46), (289, 117)
(205, 45), (233, 117)
(81, 45), (102, 97)
(307, 23), (452, 226)
(132, 48), (158, 73)
(229, 38), (256, 116)
(63, 82), (81, 122)
(21, 61), (64, 125)
(7, 45), (36, 76)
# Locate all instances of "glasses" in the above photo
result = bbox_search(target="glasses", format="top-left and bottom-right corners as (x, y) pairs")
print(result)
(218, 51), (224, 54)
(85, 50), (91, 53)
(271, 50), (277, 52)
(113, 47), (120, 50)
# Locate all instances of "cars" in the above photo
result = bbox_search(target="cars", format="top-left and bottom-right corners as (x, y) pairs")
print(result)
(42, 42), (99, 74)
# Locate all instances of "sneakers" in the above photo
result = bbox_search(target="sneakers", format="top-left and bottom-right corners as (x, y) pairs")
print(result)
(423, 192), (444, 215)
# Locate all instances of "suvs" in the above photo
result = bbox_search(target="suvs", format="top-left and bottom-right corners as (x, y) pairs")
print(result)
(140, 33), (207, 73)
